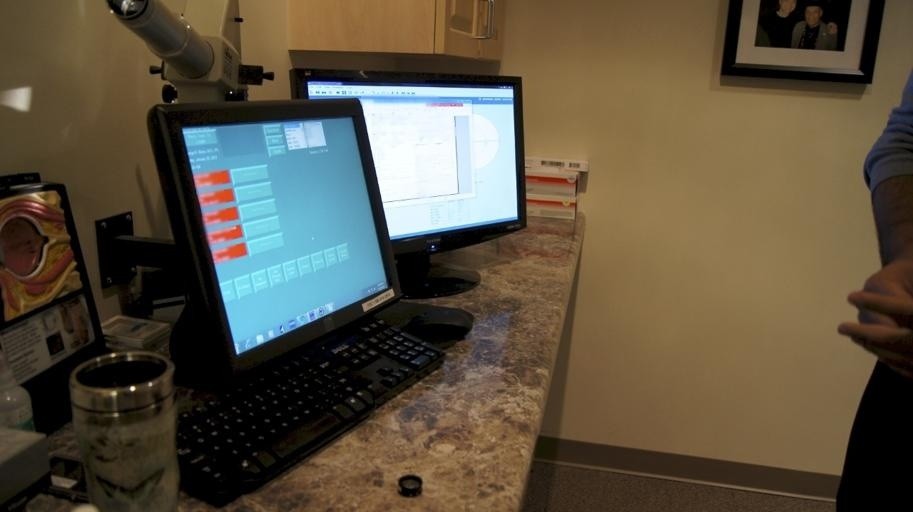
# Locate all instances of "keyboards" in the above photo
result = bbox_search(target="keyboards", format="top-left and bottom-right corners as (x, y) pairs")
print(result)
(178, 317), (445, 507)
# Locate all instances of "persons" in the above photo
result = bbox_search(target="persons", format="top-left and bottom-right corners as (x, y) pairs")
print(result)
(766, 0), (838, 48)
(836, 71), (913, 512)
(791, 5), (837, 51)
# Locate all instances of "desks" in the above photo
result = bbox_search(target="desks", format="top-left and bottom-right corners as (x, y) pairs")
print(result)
(21, 210), (583, 512)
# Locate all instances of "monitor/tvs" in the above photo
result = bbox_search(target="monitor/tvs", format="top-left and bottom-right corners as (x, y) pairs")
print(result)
(147, 98), (402, 370)
(289, 68), (527, 299)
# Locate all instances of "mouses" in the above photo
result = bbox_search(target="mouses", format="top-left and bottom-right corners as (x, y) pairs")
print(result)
(404, 307), (473, 344)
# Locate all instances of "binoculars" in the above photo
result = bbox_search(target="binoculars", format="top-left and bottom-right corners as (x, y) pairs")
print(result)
(105, 0), (275, 102)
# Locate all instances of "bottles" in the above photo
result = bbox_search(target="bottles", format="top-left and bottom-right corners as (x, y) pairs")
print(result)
(0, 352), (37, 433)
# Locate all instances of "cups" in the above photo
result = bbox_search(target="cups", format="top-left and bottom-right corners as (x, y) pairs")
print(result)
(68, 350), (181, 511)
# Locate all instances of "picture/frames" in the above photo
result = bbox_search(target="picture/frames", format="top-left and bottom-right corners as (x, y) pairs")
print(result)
(722, 0), (886, 84)
